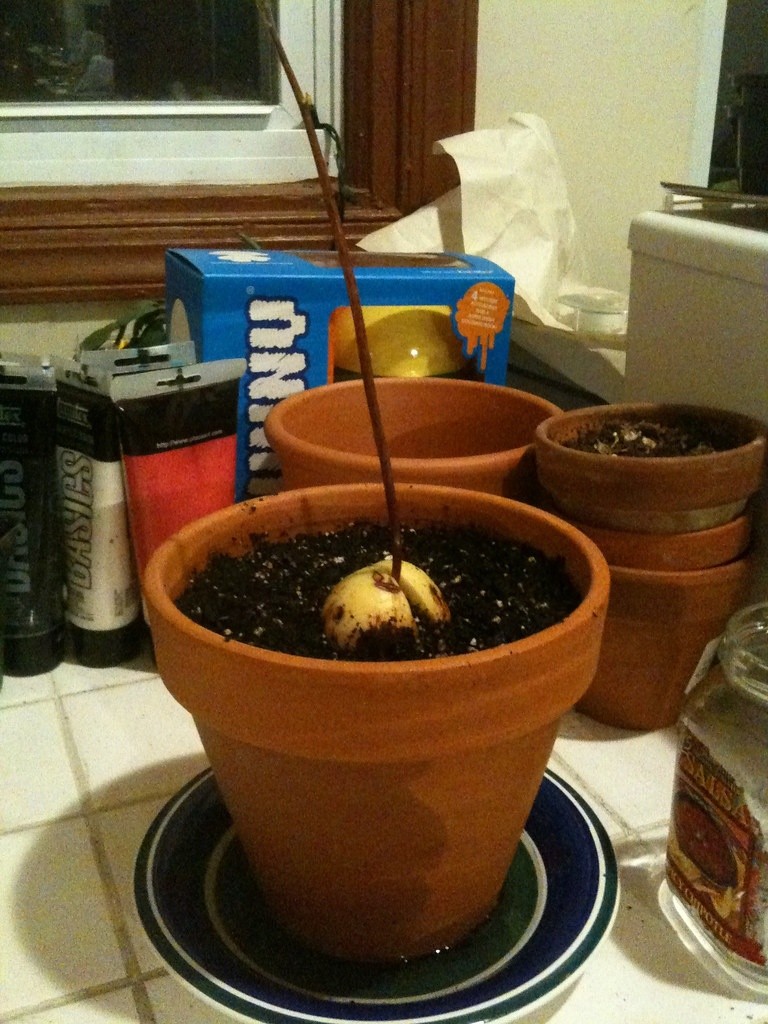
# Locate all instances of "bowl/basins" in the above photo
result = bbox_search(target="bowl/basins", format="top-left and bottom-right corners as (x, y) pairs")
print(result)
(264, 376), (566, 499)
(535, 399), (768, 730)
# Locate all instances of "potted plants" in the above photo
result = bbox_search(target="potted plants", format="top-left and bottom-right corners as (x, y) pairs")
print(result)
(141, 0), (610, 966)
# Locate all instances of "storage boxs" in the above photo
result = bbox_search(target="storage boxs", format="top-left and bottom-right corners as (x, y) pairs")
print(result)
(162, 245), (515, 512)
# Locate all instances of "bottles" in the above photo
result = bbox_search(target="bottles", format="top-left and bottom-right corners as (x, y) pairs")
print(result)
(661, 602), (767, 999)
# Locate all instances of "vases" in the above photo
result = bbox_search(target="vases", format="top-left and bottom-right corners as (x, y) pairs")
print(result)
(534, 401), (768, 730)
(264, 382), (561, 507)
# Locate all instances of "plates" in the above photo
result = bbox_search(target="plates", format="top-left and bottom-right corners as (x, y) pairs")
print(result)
(131, 748), (619, 1023)
